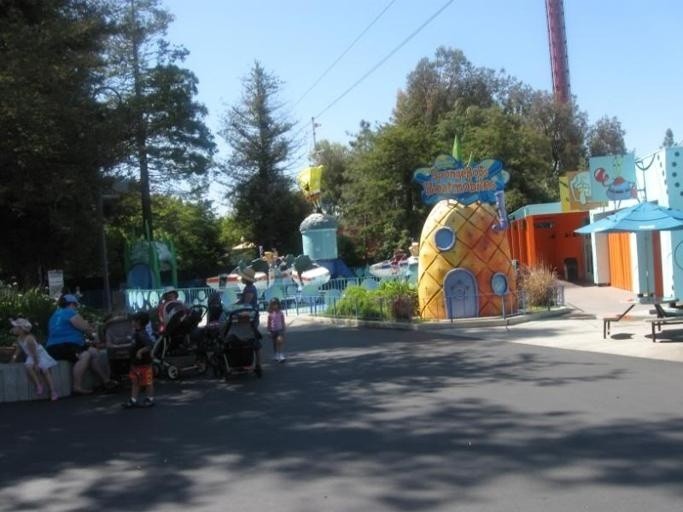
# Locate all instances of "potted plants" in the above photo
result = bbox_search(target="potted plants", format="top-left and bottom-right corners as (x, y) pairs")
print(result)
(379, 277), (418, 323)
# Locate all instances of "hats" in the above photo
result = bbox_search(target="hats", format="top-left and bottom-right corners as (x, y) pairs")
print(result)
(64, 293), (79, 304)
(240, 268), (257, 282)
(8, 317), (32, 333)
(161, 285), (178, 297)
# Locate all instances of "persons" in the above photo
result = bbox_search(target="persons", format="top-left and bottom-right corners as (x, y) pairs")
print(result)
(118, 311), (155, 408)
(45, 293), (119, 394)
(266, 297), (287, 363)
(205, 292), (226, 329)
(158, 286), (193, 332)
(81, 316), (111, 392)
(234, 268), (260, 331)
(8, 318), (58, 400)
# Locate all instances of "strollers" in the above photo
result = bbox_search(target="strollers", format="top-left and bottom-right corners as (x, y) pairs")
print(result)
(95, 312), (135, 389)
(151, 301), (209, 380)
(209, 307), (263, 382)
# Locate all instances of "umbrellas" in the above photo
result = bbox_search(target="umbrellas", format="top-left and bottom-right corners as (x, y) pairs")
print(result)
(575, 200), (682, 296)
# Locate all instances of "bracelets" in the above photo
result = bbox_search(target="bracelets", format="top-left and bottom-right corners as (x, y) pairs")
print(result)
(12, 355), (16, 358)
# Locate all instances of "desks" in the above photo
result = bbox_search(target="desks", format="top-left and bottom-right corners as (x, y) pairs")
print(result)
(609, 296), (680, 343)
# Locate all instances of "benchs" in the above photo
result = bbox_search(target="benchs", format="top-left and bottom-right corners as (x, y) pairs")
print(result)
(644, 315), (683, 342)
(603, 314), (635, 337)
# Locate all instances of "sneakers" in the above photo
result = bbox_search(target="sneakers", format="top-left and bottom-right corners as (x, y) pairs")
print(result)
(273, 352), (287, 362)
(36, 383), (44, 394)
(51, 393), (59, 401)
(120, 397), (156, 408)
